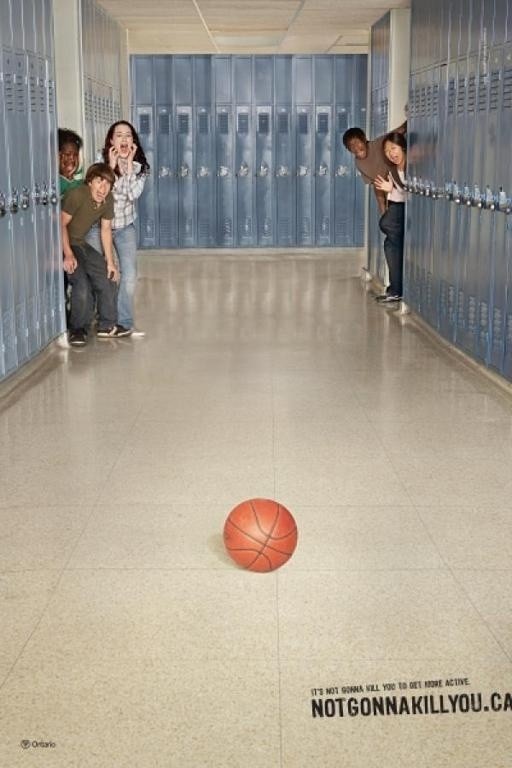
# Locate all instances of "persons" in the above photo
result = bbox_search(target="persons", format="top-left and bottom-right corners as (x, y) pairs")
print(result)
(343, 120), (407, 303)
(58, 120), (150, 346)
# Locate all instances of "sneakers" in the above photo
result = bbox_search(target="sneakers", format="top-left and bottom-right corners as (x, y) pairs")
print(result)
(376, 292), (404, 305)
(70, 325), (147, 346)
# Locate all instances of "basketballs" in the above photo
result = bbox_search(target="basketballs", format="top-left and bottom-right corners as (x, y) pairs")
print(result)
(223, 498), (298, 572)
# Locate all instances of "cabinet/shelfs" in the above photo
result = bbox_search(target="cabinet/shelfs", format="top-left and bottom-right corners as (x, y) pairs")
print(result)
(363, 7), (410, 298)
(54, 1), (131, 178)
(0, 0), (66, 408)
(128, 52), (368, 252)
(401, 0), (512, 390)
